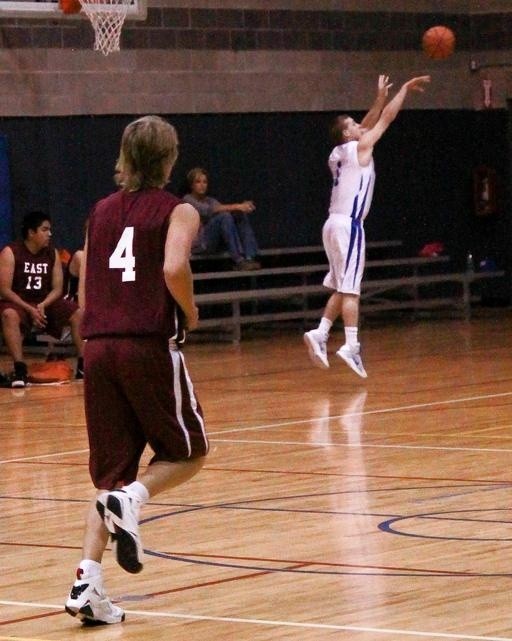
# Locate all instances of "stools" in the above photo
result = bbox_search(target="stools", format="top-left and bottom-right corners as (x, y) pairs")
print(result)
(26, 325), (71, 383)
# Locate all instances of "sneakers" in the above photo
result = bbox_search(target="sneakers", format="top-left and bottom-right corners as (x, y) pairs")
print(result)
(66, 580), (126, 625)
(75, 358), (85, 378)
(231, 256), (259, 270)
(303, 328), (330, 369)
(96, 486), (143, 573)
(11, 362), (27, 387)
(335, 343), (367, 379)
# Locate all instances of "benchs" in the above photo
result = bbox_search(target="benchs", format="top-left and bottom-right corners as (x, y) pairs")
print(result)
(181, 238), (504, 342)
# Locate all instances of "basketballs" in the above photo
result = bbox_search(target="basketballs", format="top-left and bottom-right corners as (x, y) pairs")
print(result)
(422, 25), (456, 61)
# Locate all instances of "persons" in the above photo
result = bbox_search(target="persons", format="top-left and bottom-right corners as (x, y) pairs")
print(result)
(62, 219), (89, 310)
(63, 115), (212, 624)
(303, 70), (435, 380)
(181, 168), (263, 273)
(1, 211), (85, 390)
